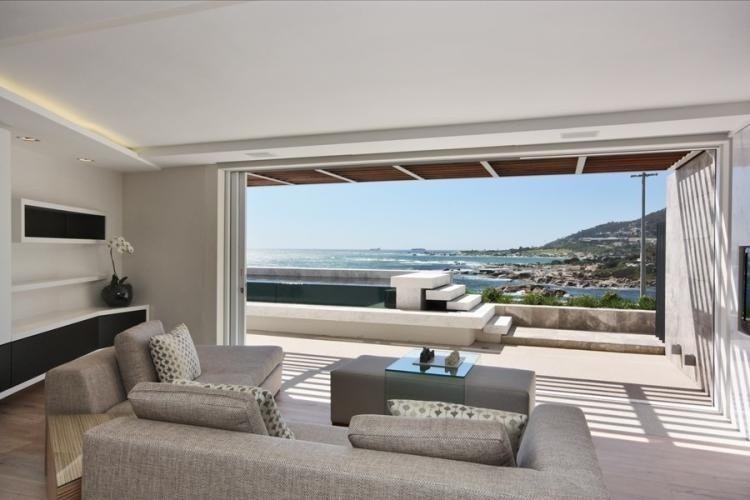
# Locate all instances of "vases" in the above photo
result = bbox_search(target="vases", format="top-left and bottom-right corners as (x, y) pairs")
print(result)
(102, 285), (132, 308)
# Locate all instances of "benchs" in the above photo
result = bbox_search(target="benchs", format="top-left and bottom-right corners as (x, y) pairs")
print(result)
(329, 355), (536, 426)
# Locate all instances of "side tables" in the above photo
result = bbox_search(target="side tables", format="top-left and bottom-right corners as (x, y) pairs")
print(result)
(45, 412), (137, 500)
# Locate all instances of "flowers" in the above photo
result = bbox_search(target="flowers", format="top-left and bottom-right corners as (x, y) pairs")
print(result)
(108, 236), (134, 284)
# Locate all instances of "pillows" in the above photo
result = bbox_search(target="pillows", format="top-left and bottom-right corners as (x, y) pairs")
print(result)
(173, 379), (295, 439)
(348, 413), (517, 467)
(385, 399), (528, 458)
(114, 320), (164, 394)
(148, 323), (202, 383)
(127, 380), (269, 435)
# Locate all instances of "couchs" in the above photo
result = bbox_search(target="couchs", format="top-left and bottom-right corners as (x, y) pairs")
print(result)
(81, 405), (608, 500)
(44, 345), (282, 418)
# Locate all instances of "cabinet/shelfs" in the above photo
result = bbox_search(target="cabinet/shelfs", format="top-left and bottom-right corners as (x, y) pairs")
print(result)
(0, 304), (149, 399)
